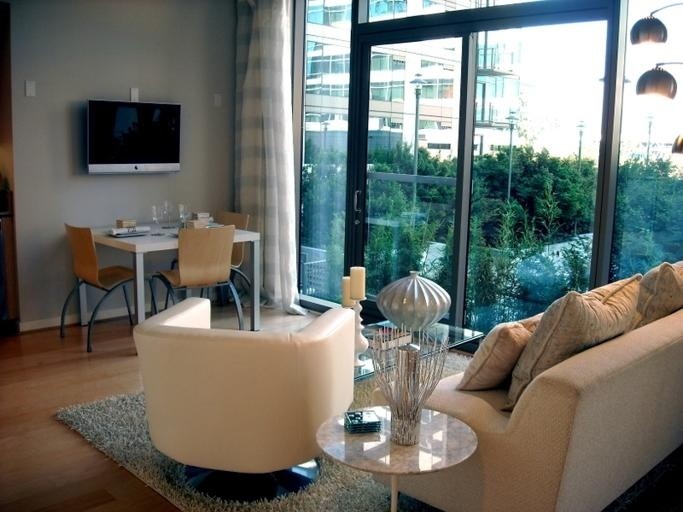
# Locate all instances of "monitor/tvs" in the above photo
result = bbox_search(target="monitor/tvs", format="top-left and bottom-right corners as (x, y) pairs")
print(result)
(86, 99), (181, 175)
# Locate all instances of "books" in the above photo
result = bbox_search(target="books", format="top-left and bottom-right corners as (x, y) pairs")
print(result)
(362, 324), (411, 351)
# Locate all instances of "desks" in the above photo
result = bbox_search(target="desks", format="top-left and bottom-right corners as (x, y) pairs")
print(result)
(79, 220), (261, 331)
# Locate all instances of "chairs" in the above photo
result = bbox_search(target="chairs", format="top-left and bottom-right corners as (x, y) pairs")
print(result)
(199, 214), (252, 312)
(148, 227), (245, 330)
(60, 223), (137, 351)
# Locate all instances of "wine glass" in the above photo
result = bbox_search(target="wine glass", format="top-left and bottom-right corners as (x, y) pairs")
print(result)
(150, 200), (186, 236)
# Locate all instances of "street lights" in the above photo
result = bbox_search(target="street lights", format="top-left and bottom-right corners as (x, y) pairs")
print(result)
(409, 71), (425, 230)
(641, 141), (659, 168)
(574, 118), (587, 236)
(504, 108), (519, 201)
(365, 163), (375, 247)
(319, 122), (330, 152)
(311, 44), (325, 123)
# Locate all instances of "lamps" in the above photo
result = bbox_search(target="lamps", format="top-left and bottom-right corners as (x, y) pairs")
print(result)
(629, 3), (683, 154)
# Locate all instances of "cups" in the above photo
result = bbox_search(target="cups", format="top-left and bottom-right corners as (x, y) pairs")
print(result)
(197, 212), (209, 228)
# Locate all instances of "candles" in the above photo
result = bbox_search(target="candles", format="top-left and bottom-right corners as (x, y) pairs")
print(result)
(342, 276), (356, 306)
(350, 266), (366, 299)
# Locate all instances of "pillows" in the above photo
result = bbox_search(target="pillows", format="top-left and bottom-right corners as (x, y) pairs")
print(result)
(455, 260), (682, 411)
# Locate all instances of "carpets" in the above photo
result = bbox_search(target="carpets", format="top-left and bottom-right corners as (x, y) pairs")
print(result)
(51, 352), (474, 512)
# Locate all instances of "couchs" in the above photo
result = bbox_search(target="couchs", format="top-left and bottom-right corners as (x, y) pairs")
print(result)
(371, 309), (683, 512)
(132, 297), (354, 499)
